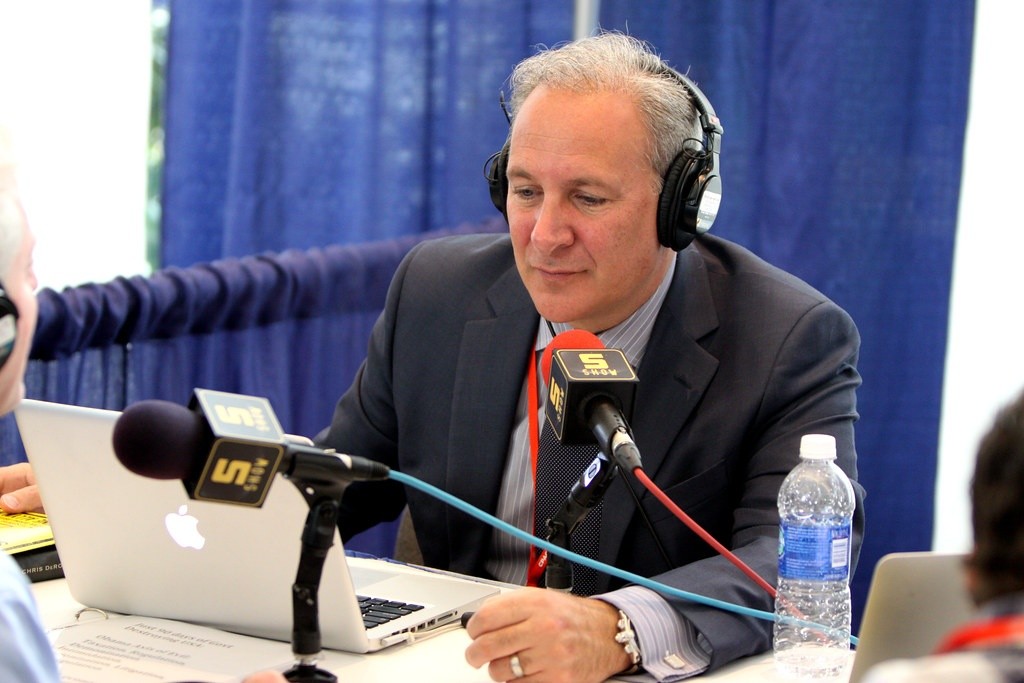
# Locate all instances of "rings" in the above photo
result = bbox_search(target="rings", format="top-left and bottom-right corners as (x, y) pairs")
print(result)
(510, 654), (524, 678)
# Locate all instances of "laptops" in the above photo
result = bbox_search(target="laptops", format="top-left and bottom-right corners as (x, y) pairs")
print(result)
(849, 553), (973, 683)
(15, 400), (503, 653)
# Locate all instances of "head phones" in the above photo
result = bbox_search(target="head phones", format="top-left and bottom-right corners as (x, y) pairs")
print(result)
(484, 60), (724, 252)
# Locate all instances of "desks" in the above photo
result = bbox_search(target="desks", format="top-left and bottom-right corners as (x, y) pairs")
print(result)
(28, 548), (854, 683)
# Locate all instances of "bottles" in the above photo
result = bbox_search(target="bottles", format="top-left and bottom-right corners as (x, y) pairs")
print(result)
(773, 434), (856, 678)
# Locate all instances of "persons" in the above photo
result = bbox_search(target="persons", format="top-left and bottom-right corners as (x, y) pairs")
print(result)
(0, 21), (868, 683)
(0, 163), (64, 683)
(862, 383), (1024, 683)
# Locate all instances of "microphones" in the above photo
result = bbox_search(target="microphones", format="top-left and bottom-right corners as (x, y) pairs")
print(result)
(499, 90), (512, 126)
(541, 329), (643, 474)
(113, 388), (392, 507)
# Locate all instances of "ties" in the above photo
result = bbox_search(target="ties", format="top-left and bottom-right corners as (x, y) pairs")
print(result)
(535, 415), (604, 598)
